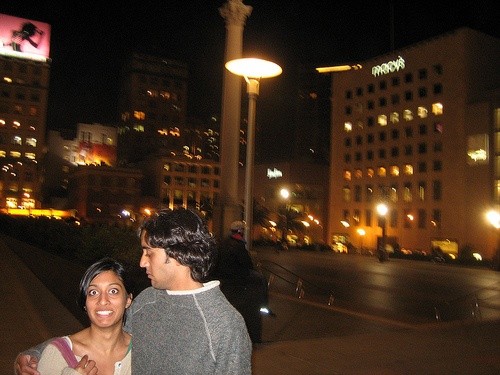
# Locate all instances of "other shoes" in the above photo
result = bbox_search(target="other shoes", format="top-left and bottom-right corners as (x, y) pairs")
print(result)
(259, 306), (277, 318)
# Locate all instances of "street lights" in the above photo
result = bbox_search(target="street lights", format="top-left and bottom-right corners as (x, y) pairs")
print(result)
(374, 202), (392, 249)
(224, 56), (282, 254)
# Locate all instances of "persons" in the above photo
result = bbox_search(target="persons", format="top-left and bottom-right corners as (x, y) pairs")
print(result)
(37, 257), (133, 375)
(14, 207), (252, 375)
(223, 220), (277, 317)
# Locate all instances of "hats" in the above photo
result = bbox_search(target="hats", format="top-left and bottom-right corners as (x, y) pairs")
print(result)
(230, 221), (246, 229)
